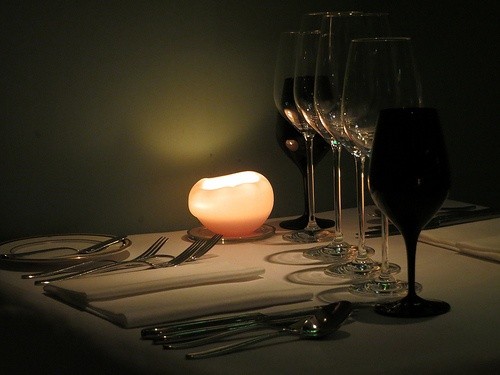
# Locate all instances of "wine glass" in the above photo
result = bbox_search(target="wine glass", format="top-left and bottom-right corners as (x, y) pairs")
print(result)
(273, 29), (337, 245)
(275, 74), (336, 231)
(293, 12), (375, 262)
(366, 106), (452, 319)
(312, 13), (402, 277)
(340, 35), (424, 299)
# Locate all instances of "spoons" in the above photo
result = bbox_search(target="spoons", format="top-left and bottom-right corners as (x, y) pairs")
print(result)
(185, 300), (355, 360)
(366, 204), (478, 218)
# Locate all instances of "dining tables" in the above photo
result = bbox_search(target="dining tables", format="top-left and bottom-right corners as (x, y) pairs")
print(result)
(0, 204), (500, 375)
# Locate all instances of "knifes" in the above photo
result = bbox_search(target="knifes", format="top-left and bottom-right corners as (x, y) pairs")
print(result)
(140, 301), (382, 350)
(356, 206), (500, 238)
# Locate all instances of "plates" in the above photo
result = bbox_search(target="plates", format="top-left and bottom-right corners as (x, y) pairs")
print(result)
(0, 233), (132, 267)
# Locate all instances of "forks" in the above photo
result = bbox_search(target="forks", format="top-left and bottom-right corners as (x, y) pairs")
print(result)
(34, 238), (206, 286)
(62, 233), (224, 274)
(0, 234), (128, 258)
(21, 235), (169, 279)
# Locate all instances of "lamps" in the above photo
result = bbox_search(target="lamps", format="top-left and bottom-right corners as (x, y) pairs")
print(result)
(188, 170), (276, 243)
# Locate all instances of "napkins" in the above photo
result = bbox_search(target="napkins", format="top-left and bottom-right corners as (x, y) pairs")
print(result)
(418, 219), (500, 262)
(44, 257), (313, 329)
